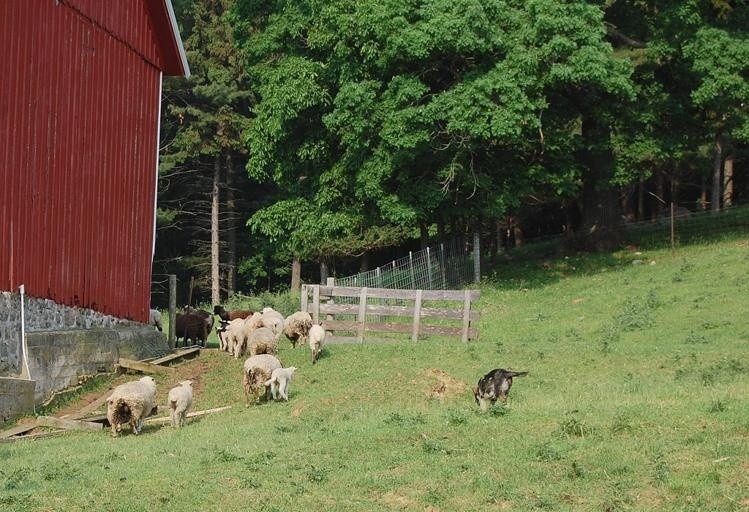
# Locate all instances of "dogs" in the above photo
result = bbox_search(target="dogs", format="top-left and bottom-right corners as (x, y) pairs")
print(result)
(473, 369), (529, 409)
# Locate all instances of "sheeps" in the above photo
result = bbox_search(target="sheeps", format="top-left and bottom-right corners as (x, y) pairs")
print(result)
(308, 322), (325, 364)
(265, 366), (298, 401)
(148, 309), (163, 331)
(105, 377), (157, 438)
(243, 354), (283, 408)
(167, 380), (193, 428)
(175, 306), (312, 359)
